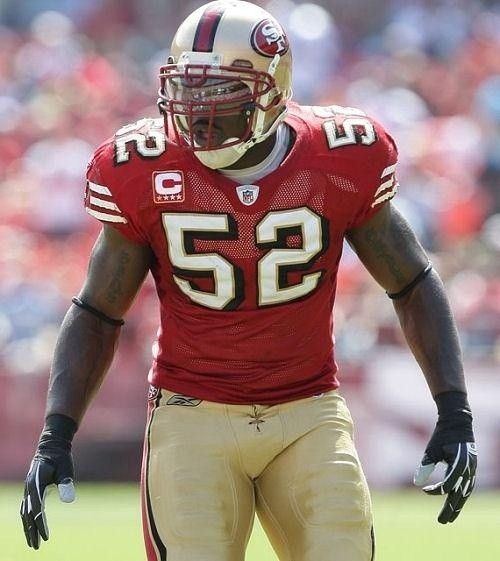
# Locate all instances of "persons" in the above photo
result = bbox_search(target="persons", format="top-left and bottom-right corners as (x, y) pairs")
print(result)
(20, 1), (478, 561)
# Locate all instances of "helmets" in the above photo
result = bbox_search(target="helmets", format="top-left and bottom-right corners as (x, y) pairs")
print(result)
(157, 2), (292, 169)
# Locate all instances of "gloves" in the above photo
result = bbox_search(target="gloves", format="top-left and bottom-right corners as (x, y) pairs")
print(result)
(413, 392), (477, 524)
(21, 415), (78, 550)
